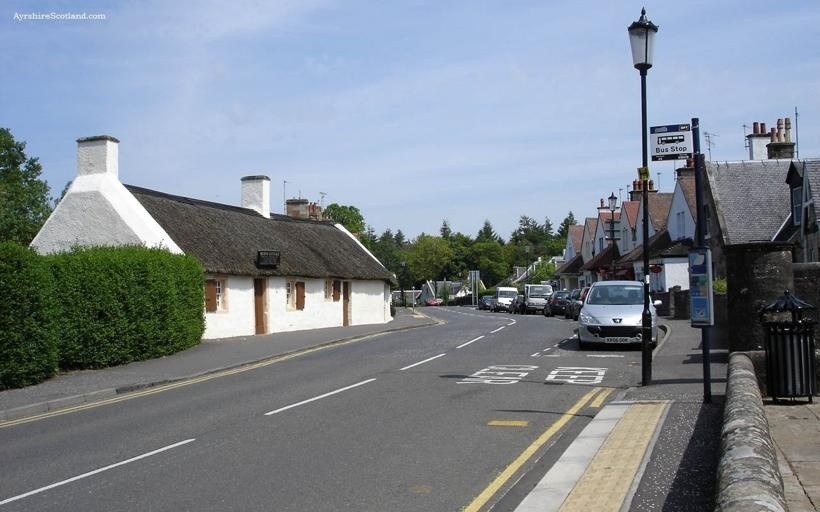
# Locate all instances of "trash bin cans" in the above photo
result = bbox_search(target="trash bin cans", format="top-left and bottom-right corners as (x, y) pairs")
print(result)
(759, 288), (816, 405)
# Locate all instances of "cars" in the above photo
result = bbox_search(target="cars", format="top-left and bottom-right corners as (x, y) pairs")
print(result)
(479, 280), (662, 348)
(423, 298), (440, 306)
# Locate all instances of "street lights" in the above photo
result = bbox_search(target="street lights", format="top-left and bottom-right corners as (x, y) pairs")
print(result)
(524, 242), (532, 279)
(627, 6), (659, 386)
(607, 192), (617, 278)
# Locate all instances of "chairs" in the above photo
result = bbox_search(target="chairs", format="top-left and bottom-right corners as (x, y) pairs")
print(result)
(596, 288), (611, 304)
(626, 290), (640, 303)
